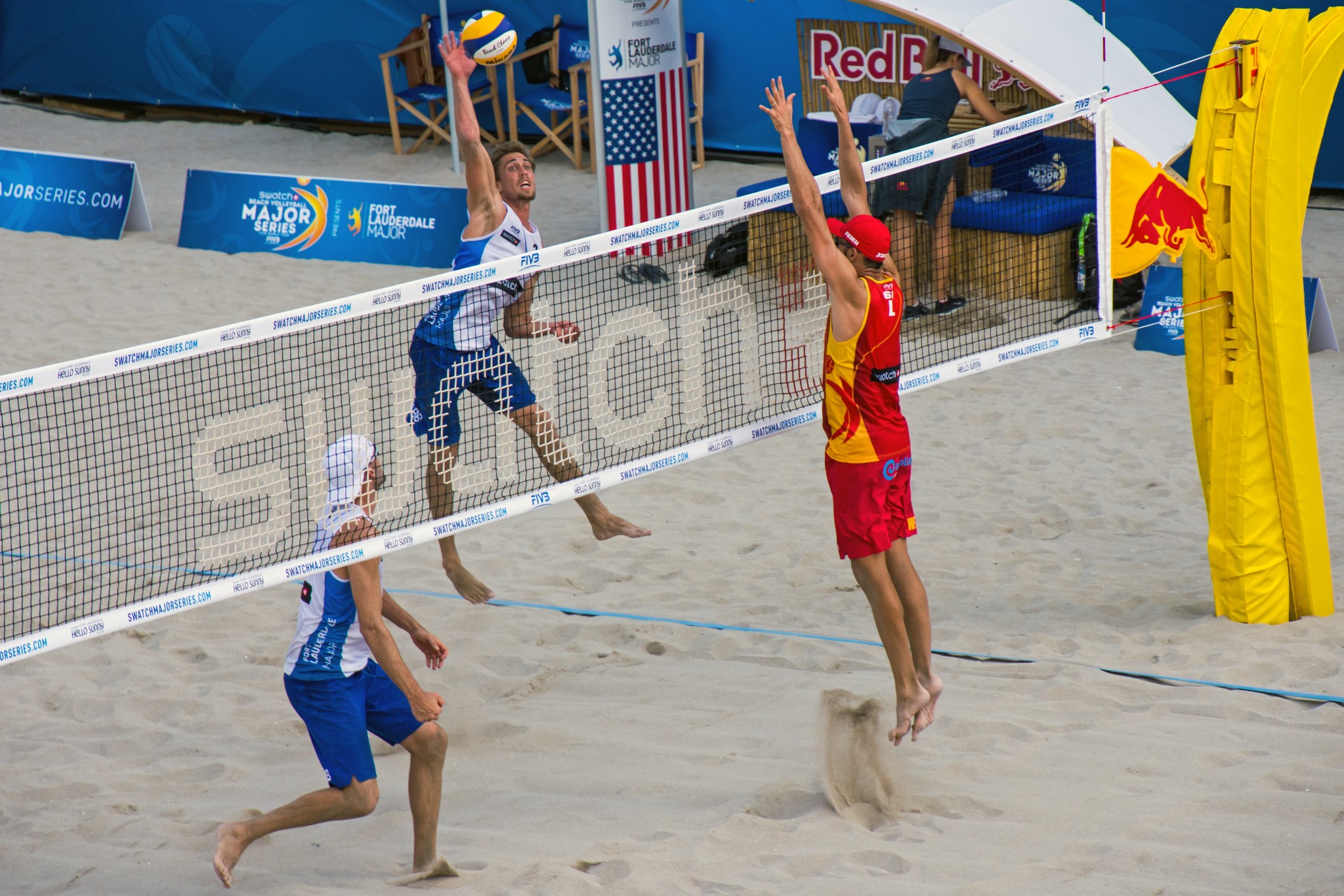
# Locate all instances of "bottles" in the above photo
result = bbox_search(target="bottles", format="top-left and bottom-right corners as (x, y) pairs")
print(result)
(971, 188), (1008, 203)
(882, 96), (896, 135)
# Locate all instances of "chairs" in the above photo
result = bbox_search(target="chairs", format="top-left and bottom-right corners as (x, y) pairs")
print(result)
(736, 117), (884, 218)
(375, 12), (711, 176)
(951, 134), (1097, 236)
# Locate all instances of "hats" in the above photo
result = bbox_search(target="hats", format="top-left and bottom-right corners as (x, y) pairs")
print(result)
(322, 434), (373, 505)
(826, 215), (891, 262)
(933, 34), (972, 67)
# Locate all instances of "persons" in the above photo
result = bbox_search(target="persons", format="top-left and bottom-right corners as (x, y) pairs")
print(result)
(212, 437), (450, 889)
(757, 65), (940, 747)
(872, 34), (1018, 323)
(405, 30), (654, 603)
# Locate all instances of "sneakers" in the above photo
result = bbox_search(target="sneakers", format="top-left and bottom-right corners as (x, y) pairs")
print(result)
(935, 290), (966, 316)
(905, 297), (930, 320)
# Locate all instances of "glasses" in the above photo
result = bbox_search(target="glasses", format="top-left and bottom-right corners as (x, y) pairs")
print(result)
(835, 237), (862, 253)
(365, 444), (380, 470)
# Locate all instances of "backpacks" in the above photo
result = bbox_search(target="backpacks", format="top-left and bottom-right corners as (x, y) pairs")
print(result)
(395, 15), (434, 89)
(522, 21), (565, 85)
(1071, 213), (1146, 311)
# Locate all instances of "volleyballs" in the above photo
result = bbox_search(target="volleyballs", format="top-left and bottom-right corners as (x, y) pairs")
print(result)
(461, 10), (518, 67)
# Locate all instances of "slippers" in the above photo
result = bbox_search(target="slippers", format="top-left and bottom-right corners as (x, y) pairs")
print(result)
(638, 263), (671, 284)
(618, 264), (644, 283)
